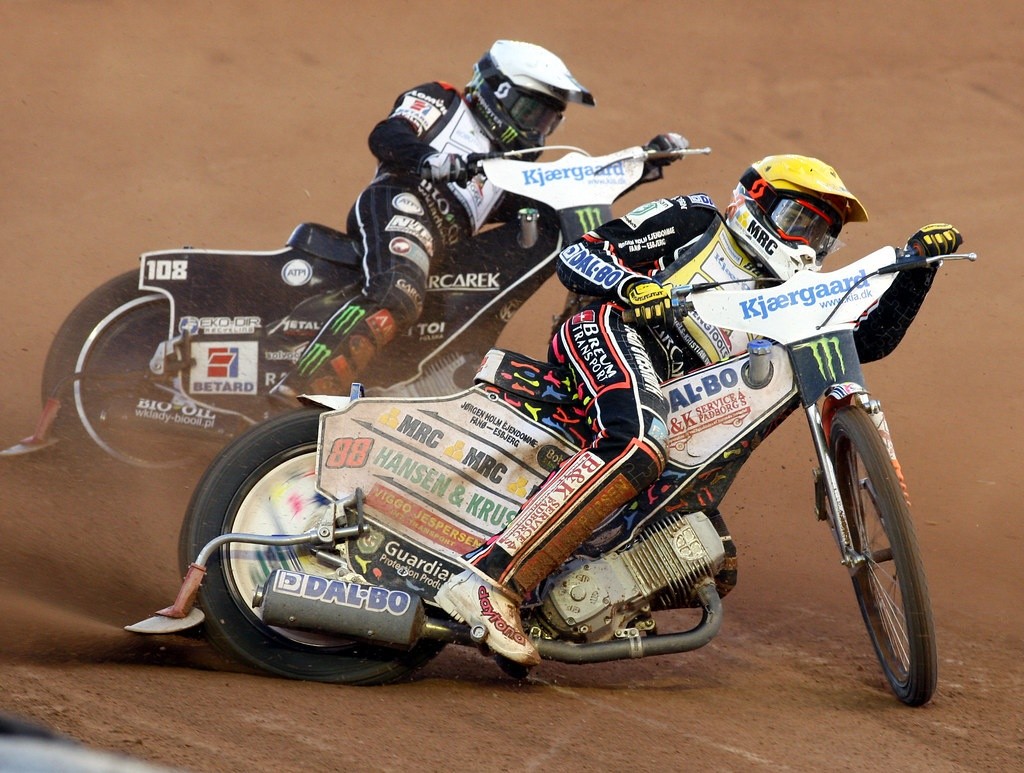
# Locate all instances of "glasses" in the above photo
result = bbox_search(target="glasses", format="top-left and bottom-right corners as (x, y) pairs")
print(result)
(741, 167), (833, 256)
(479, 54), (563, 136)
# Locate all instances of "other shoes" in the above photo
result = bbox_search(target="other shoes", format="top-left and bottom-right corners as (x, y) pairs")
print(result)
(264, 374), (316, 414)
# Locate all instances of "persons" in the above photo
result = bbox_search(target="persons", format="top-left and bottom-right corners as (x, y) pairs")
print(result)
(438, 154), (965, 672)
(266, 40), (688, 422)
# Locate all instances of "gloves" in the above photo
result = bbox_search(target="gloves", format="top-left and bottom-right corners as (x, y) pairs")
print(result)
(905, 222), (963, 268)
(423, 153), (468, 190)
(646, 131), (688, 167)
(627, 279), (675, 330)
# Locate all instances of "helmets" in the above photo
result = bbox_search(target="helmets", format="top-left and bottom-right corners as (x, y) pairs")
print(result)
(466, 40), (596, 160)
(725, 154), (869, 284)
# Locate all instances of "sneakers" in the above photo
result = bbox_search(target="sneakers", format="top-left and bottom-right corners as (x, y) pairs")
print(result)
(434, 569), (539, 666)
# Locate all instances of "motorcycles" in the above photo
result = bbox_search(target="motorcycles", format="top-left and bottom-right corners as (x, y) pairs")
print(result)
(117, 233), (979, 707)
(0, 136), (713, 488)
